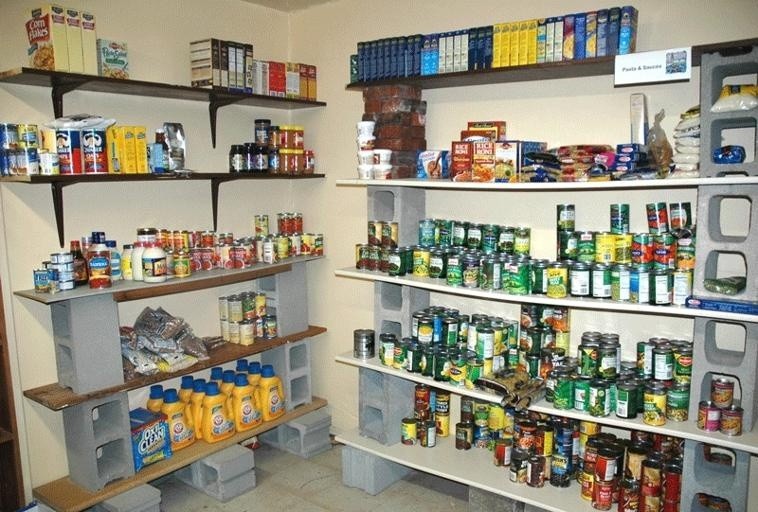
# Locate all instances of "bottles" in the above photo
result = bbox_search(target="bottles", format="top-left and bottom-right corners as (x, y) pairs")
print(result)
(134, 357), (288, 451)
(227, 116), (314, 175)
(67, 228), (169, 284)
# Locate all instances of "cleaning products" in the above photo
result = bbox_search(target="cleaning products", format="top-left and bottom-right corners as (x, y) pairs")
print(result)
(178, 375), (193, 402)
(201, 382), (236, 444)
(219, 369), (235, 395)
(209, 365), (223, 390)
(247, 362), (261, 385)
(147, 384), (164, 414)
(258, 365), (286, 423)
(189, 378), (206, 439)
(160, 388), (196, 452)
(236, 358), (247, 373)
(232, 373), (263, 432)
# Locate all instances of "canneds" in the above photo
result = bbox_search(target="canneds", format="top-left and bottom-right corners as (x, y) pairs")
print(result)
(354, 301), (693, 426)
(228, 119), (314, 174)
(354, 202), (695, 307)
(1, 123), (62, 177)
(697, 378), (744, 438)
(219, 291), (277, 346)
(402, 380), (449, 448)
(156, 213), (322, 278)
(456, 384), (731, 512)
